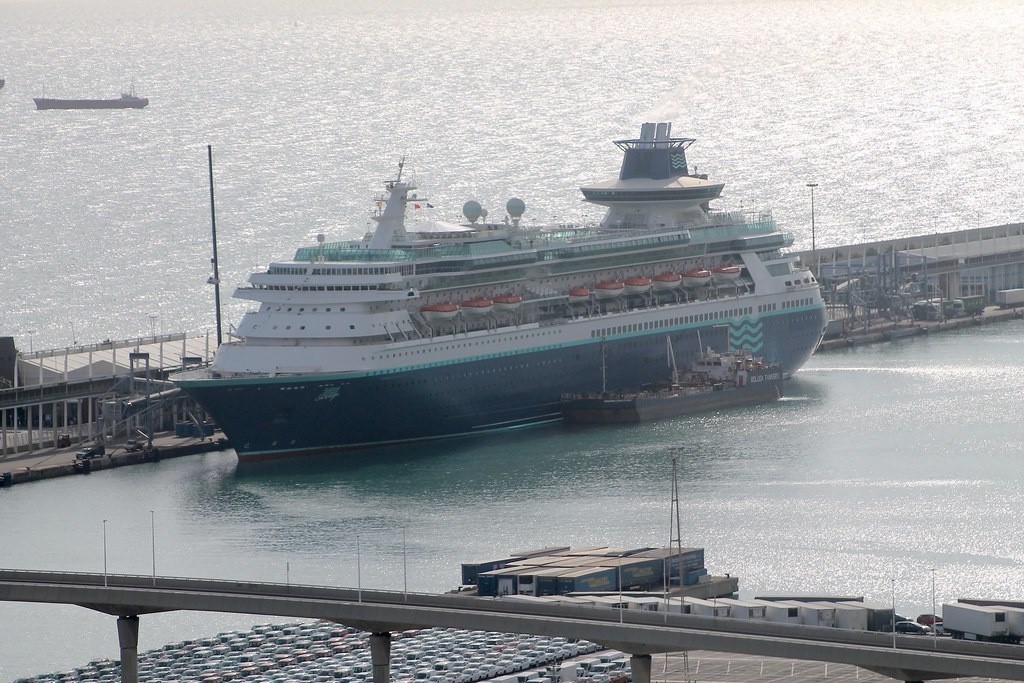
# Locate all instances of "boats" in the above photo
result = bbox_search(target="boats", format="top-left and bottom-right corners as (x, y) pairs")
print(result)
(0, 78), (6, 89)
(556, 322), (783, 426)
(32, 74), (149, 110)
(168, 122), (831, 475)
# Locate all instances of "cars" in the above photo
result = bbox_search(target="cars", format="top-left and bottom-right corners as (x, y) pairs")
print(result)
(76, 445), (105, 458)
(895, 621), (930, 635)
(917, 613), (943, 626)
(8, 620), (631, 682)
(58, 434), (71, 447)
(930, 622), (952, 633)
(895, 613), (912, 622)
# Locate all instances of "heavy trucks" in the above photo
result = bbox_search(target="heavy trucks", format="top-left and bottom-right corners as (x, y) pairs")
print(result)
(913, 297), (948, 321)
(952, 294), (986, 317)
(927, 300), (954, 320)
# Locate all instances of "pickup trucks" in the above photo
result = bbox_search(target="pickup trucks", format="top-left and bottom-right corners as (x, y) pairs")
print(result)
(126, 439), (143, 451)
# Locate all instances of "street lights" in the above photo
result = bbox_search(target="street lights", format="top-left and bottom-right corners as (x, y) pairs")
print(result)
(400, 524), (407, 603)
(974, 211), (983, 261)
(150, 510), (155, 585)
(931, 216), (938, 267)
(930, 568), (937, 649)
(618, 554), (622, 622)
(357, 534), (361, 603)
(891, 579), (896, 647)
(103, 519), (108, 588)
(67, 322), (76, 348)
(661, 545), (667, 623)
(858, 228), (869, 270)
(805, 182), (819, 268)
